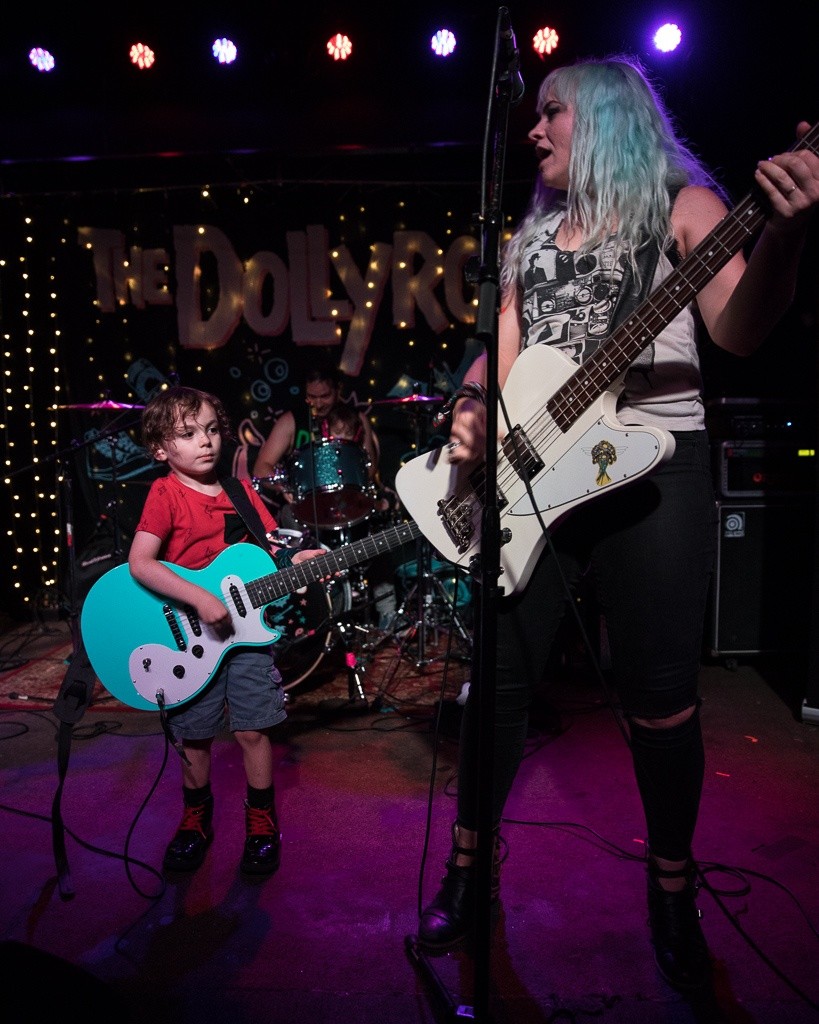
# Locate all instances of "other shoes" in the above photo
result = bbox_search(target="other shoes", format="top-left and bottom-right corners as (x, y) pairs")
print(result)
(378, 615), (410, 638)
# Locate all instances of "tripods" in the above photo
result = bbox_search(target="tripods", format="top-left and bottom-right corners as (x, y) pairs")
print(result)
(368, 540), (476, 674)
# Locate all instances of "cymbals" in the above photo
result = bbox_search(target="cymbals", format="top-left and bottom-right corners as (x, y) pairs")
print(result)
(47, 399), (146, 412)
(356, 394), (445, 406)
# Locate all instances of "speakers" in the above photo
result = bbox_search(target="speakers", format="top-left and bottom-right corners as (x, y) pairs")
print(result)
(703, 500), (776, 659)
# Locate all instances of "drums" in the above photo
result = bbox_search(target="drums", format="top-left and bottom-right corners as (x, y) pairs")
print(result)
(282, 437), (378, 528)
(268, 528), (353, 692)
(329, 523), (397, 588)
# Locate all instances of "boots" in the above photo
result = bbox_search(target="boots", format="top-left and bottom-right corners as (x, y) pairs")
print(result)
(417, 823), (511, 953)
(241, 784), (282, 875)
(160, 781), (216, 883)
(643, 845), (717, 992)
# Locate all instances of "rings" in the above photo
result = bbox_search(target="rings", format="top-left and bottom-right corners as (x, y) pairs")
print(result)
(785, 183), (799, 199)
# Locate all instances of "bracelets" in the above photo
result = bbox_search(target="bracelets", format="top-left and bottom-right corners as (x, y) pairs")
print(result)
(432, 382), (487, 428)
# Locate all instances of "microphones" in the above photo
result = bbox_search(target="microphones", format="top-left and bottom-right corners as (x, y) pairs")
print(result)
(312, 403), (324, 440)
(496, 8), (525, 101)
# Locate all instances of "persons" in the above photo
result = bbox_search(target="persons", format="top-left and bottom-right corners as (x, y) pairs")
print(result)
(254, 365), (417, 637)
(127, 386), (334, 879)
(417, 59), (818, 981)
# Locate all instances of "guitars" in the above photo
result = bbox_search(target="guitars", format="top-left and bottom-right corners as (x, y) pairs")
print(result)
(393, 123), (819, 597)
(80, 521), (422, 711)
